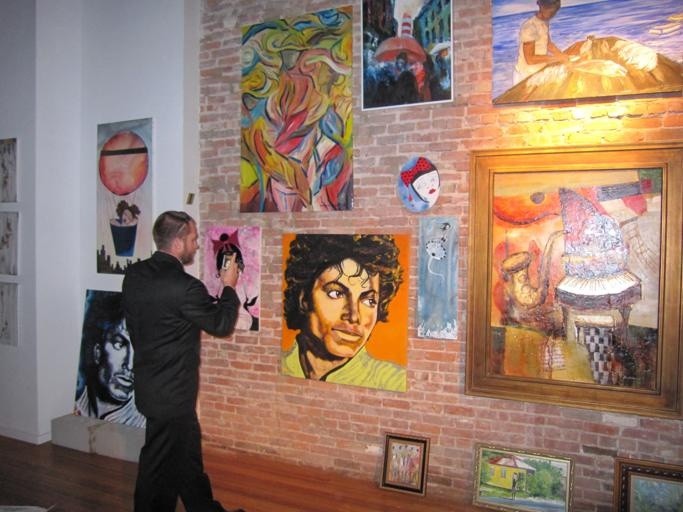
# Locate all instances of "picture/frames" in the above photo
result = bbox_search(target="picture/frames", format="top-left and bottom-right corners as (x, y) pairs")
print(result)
(377, 432), (430, 496)
(464, 139), (683, 421)
(472, 443), (576, 512)
(613, 456), (683, 512)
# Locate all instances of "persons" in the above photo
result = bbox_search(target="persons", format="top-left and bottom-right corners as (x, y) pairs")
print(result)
(512, 0), (569, 88)
(74, 291), (147, 429)
(280, 235), (408, 392)
(123, 210), (239, 511)
